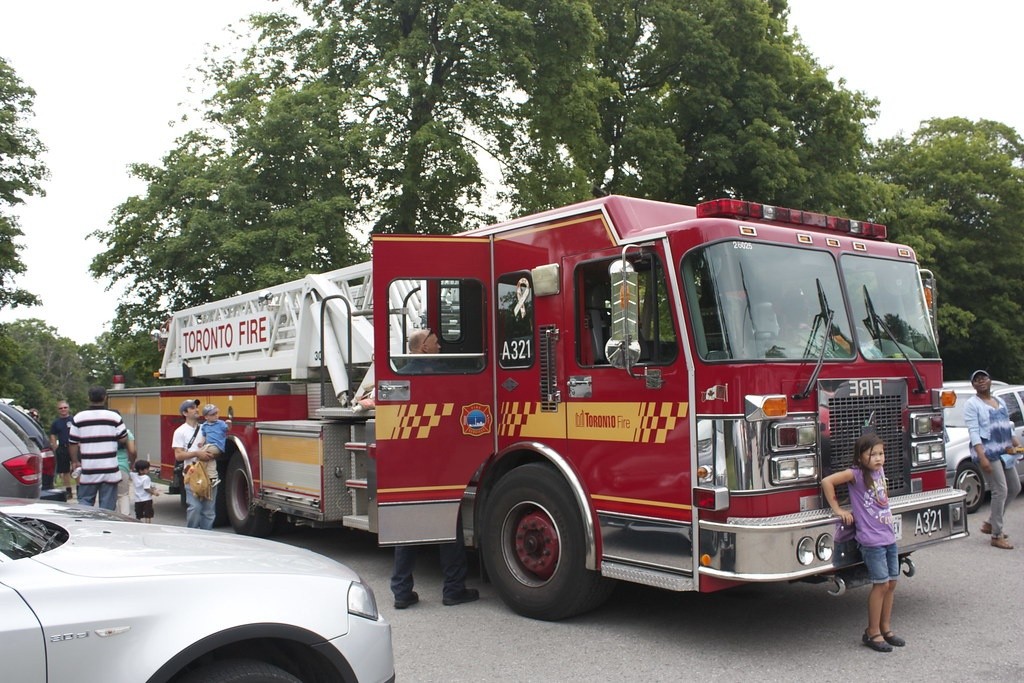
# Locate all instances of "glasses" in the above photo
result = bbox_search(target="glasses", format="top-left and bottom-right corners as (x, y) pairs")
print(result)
(423, 328), (433, 344)
(59, 407), (67, 410)
(33, 414), (39, 418)
(972, 376), (988, 382)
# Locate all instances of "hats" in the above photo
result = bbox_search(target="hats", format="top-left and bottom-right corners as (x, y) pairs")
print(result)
(202, 404), (218, 416)
(971, 369), (990, 383)
(180, 398), (200, 413)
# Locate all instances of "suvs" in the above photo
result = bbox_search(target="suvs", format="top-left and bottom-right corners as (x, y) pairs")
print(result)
(0, 397), (77, 502)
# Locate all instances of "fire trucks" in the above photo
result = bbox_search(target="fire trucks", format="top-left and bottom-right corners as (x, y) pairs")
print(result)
(107, 193), (970, 624)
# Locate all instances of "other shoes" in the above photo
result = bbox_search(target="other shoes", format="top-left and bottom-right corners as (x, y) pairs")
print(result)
(443, 588), (479, 605)
(66, 491), (72, 499)
(883, 631), (905, 646)
(394, 591), (418, 608)
(862, 628), (893, 652)
(980, 522), (1008, 539)
(990, 536), (1014, 549)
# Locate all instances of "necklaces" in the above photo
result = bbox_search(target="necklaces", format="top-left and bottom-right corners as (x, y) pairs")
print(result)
(873, 468), (888, 505)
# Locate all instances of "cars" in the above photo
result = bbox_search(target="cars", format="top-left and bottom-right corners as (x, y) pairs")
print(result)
(0, 494), (143, 525)
(0, 509), (396, 683)
(945, 384), (1024, 515)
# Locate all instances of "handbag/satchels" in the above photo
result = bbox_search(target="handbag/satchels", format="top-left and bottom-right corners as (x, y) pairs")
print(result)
(172, 462), (184, 493)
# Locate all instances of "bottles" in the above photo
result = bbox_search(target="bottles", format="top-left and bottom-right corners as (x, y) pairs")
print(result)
(71, 466), (82, 479)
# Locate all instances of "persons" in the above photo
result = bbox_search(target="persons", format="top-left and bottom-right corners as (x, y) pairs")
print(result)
(29, 408), (41, 422)
(109, 409), (137, 516)
(389, 328), (479, 609)
(963, 370), (1024, 549)
(821, 431), (906, 652)
(130, 460), (160, 523)
(172, 399), (232, 530)
(50, 399), (81, 498)
(67, 384), (128, 511)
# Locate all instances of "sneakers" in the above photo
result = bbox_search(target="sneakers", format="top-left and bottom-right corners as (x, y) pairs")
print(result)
(210, 478), (221, 488)
